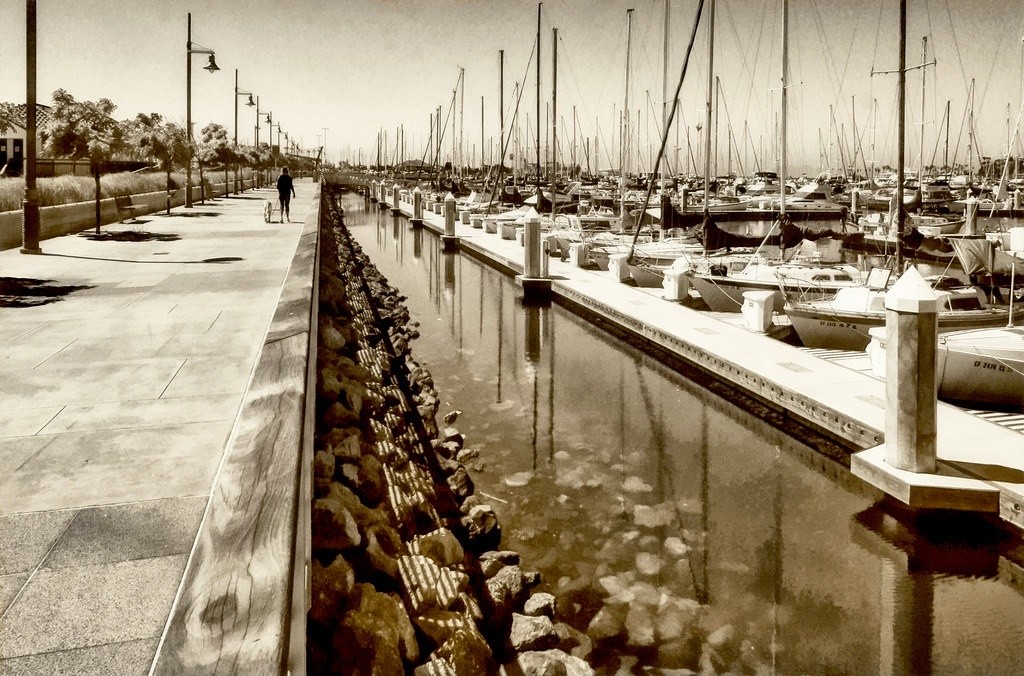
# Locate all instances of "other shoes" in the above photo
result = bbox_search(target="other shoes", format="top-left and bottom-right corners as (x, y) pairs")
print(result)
(280, 220), (284, 223)
(287, 218), (290, 223)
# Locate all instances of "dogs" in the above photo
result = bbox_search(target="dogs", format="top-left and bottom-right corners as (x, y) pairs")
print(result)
(264, 202), (272, 223)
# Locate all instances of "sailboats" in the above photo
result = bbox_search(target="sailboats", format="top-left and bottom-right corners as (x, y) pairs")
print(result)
(364, 1), (1023, 411)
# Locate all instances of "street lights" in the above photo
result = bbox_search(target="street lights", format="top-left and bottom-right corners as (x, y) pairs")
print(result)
(233, 69), (256, 195)
(186, 10), (220, 207)
(255, 95), (302, 191)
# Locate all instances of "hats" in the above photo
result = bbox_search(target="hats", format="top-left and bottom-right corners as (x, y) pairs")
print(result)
(282, 168), (288, 173)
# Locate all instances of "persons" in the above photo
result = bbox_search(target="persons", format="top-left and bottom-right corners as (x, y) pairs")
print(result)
(970, 237), (1009, 305)
(276, 166), (296, 223)
